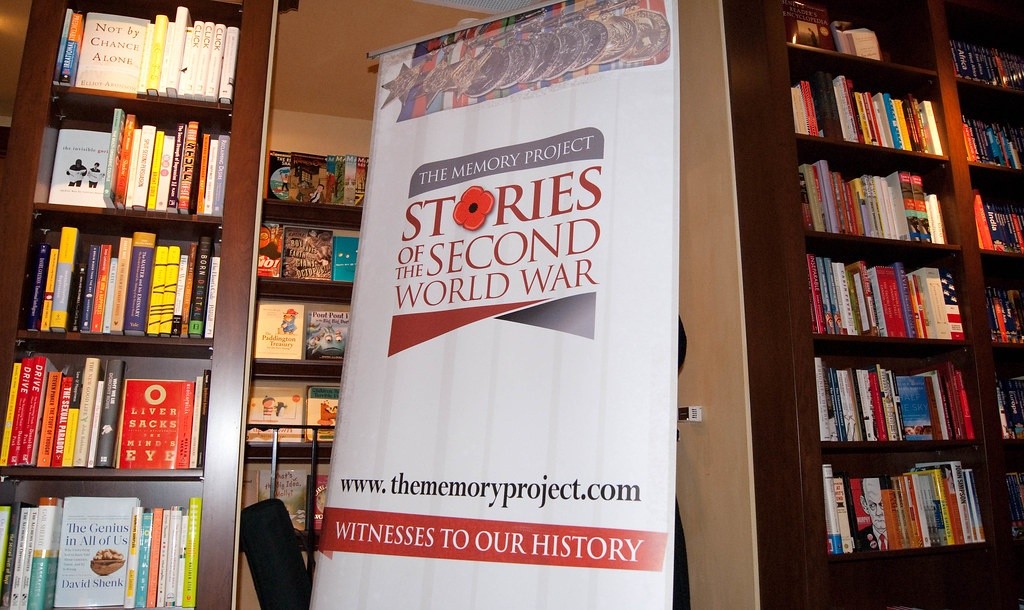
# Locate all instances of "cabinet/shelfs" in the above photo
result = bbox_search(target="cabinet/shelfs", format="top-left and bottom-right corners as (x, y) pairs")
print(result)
(0, 0), (1024, 610)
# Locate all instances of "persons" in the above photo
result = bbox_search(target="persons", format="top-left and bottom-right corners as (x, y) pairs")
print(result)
(67, 159), (87, 187)
(88, 163), (105, 188)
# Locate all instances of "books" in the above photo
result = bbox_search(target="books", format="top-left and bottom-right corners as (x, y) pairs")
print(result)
(19, 212), (223, 340)
(791, 72), (943, 156)
(806, 253), (966, 340)
(962, 113), (1024, 169)
(950, 39), (1024, 91)
(799, 160), (948, 245)
(267, 149), (370, 207)
(814, 357), (975, 442)
(982, 285), (1024, 610)
(973, 188), (1024, 253)
(821, 461), (986, 554)
(48, 106), (229, 216)
(254, 298), (350, 360)
(246, 379), (341, 447)
(0, 496), (202, 610)
(53, 6), (240, 105)
(259, 220), (359, 282)
(782, 0), (882, 61)
(0, 355), (210, 470)
(242, 468), (328, 530)
